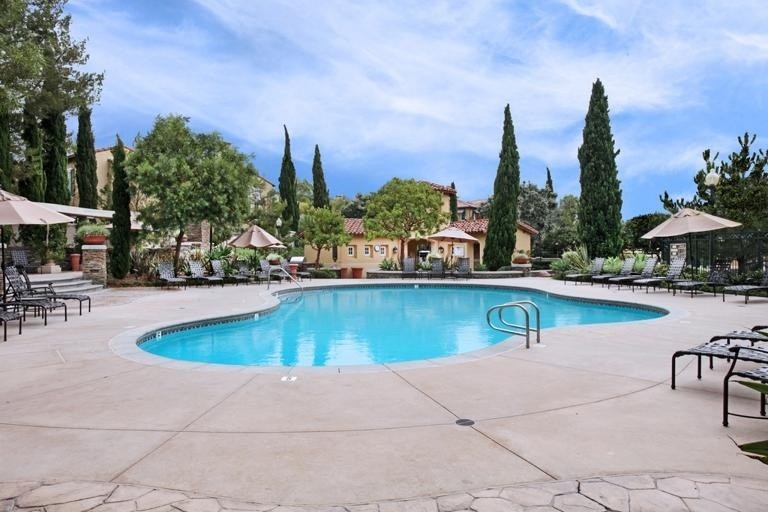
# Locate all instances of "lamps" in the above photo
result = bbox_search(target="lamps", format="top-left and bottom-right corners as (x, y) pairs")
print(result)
(393, 246), (398, 253)
(438, 246), (444, 254)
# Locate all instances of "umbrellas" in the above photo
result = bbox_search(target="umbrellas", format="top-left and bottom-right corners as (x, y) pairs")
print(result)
(0, 190), (76, 314)
(227, 226), (283, 274)
(425, 226), (480, 278)
(268, 244), (287, 249)
(637, 209), (745, 296)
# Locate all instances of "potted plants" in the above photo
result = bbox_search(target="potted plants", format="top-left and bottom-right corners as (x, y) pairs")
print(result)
(76, 224), (112, 245)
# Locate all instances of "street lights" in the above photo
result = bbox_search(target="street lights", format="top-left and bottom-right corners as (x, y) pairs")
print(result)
(275, 217), (282, 280)
(705, 169), (719, 281)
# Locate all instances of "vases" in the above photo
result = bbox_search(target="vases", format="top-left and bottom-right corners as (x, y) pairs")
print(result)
(70, 253), (80, 270)
(351, 267), (363, 278)
(339, 268), (347, 278)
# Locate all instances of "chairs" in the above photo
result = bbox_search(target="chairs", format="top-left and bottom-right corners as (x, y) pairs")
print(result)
(563, 255), (768, 304)
(427, 255), (447, 279)
(670, 323), (768, 435)
(156, 257), (313, 289)
(453, 256), (472, 278)
(8, 250), (42, 273)
(401, 256), (418, 280)
(0, 264), (93, 344)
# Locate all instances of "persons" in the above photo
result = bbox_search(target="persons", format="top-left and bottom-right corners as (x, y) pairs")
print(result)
(436, 246), (445, 258)
(517, 249), (529, 263)
(266, 247), (283, 265)
